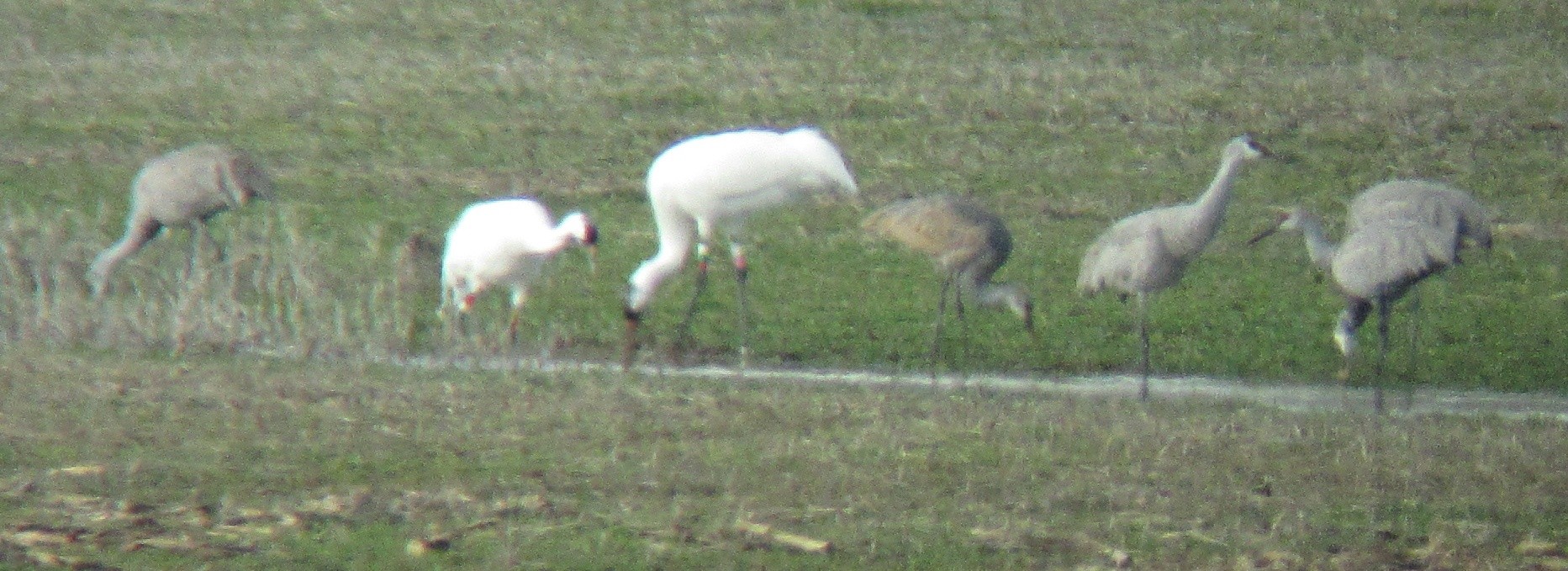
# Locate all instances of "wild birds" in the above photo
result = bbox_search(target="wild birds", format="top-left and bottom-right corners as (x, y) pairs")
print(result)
(1073, 136), (1280, 402)
(831, 193), (1034, 397)
(88, 143), (272, 300)
(620, 129), (861, 379)
(440, 196), (599, 358)
(1247, 178), (1495, 379)
